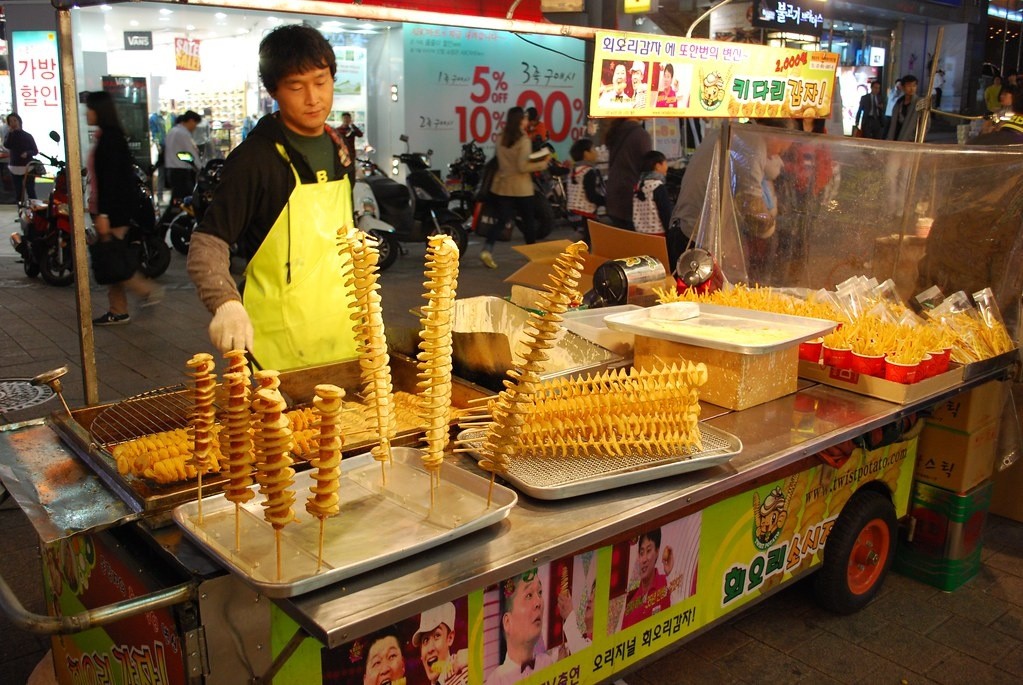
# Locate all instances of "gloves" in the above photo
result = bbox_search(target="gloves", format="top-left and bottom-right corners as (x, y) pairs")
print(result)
(209, 299), (253, 355)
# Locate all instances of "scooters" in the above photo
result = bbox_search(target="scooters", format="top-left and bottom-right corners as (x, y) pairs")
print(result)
(445, 138), (590, 242)
(354, 166), (405, 272)
(359, 135), (469, 263)
(131, 158), (173, 278)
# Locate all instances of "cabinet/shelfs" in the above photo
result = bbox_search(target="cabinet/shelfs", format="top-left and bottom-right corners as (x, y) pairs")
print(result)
(211, 126), (231, 153)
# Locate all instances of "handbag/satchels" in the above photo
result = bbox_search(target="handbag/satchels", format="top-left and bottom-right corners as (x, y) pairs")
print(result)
(27, 156), (47, 176)
(88, 231), (138, 286)
(852, 126), (863, 137)
(472, 192), (514, 242)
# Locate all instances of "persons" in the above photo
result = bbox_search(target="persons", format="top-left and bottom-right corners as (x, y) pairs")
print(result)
(336, 112), (363, 166)
(604, 116), (652, 233)
(933, 69), (946, 109)
(902, 73), (1023, 341)
(886, 75), (930, 217)
(359, 602), (469, 685)
(150, 107), (177, 192)
(656, 63), (678, 107)
(668, 116), (833, 288)
(599, 61), (646, 107)
(566, 139), (607, 254)
(83, 91), (158, 327)
(631, 150), (678, 238)
(155, 111), (203, 245)
(477, 106), (550, 269)
(854, 81), (886, 141)
(884, 78), (905, 140)
(484, 570), (594, 685)
(191, 110), (211, 158)
(0, 112), (39, 221)
(187, 24), (368, 374)
(621, 527), (684, 629)
(524, 106), (550, 143)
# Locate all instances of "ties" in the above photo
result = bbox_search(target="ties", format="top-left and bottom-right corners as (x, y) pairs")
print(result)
(873, 95), (877, 120)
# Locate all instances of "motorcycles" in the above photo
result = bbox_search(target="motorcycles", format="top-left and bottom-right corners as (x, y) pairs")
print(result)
(10, 131), (92, 288)
(167, 153), (228, 256)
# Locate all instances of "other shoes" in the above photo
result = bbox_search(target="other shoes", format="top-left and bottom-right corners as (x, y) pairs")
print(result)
(478, 249), (498, 269)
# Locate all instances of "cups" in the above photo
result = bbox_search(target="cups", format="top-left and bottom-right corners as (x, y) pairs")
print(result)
(799, 323), (953, 384)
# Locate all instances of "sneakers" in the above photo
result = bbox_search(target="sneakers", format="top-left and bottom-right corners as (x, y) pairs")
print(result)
(139, 284), (172, 310)
(92, 311), (132, 326)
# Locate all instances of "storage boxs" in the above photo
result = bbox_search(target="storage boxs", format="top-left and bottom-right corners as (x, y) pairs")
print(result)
(504, 219), (674, 316)
(890, 371), (1014, 592)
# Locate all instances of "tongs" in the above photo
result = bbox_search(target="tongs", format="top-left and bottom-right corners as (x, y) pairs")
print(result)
(244, 346), (294, 408)
